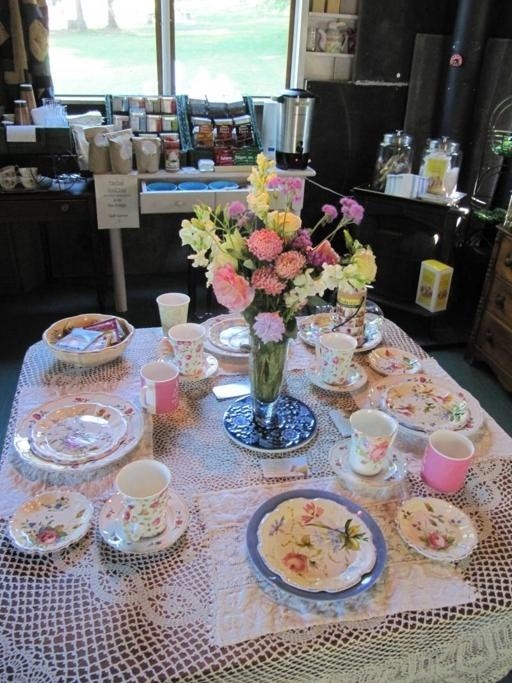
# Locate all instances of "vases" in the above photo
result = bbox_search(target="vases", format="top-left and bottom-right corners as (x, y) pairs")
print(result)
(247, 304), (287, 425)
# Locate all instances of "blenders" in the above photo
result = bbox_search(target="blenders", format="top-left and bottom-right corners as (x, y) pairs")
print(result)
(271, 88), (321, 171)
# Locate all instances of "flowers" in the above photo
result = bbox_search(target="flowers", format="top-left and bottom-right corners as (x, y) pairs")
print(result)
(172, 155), (377, 426)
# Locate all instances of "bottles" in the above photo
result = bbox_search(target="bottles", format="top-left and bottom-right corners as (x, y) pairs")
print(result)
(372, 129), (415, 192)
(13, 98), (31, 125)
(306, 11), (318, 51)
(419, 135), (463, 200)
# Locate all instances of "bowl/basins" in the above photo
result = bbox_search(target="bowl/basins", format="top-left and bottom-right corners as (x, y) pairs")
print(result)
(0, 164), (87, 194)
(145, 180), (241, 193)
(43, 314), (135, 369)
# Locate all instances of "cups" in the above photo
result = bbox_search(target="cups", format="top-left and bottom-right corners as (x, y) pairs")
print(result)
(421, 429), (477, 495)
(3, 112), (15, 121)
(348, 409), (398, 476)
(20, 82), (37, 109)
(313, 331), (357, 386)
(139, 361), (181, 414)
(167, 322), (204, 374)
(114, 457), (175, 542)
(156, 291), (191, 335)
(163, 139), (180, 173)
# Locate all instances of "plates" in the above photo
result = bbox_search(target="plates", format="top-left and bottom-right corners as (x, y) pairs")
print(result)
(366, 374), (483, 441)
(367, 348), (424, 375)
(257, 495), (377, 593)
(299, 316), (383, 353)
(12, 391), (144, 473)
(199, 312), (260, 359)
(8, 487), (93, 554)
(28, 402), (128, 464)
(393, 496), (479, 562)
(157, 352), (219, 382)
(302, 359), (370, 394)
(98, 487), (188, 554)
(328, 438), (410, 487)
(245, 489), (387, 599)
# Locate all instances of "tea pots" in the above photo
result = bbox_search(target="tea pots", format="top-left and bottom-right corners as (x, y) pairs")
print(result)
(316, 20), (349, 53)
